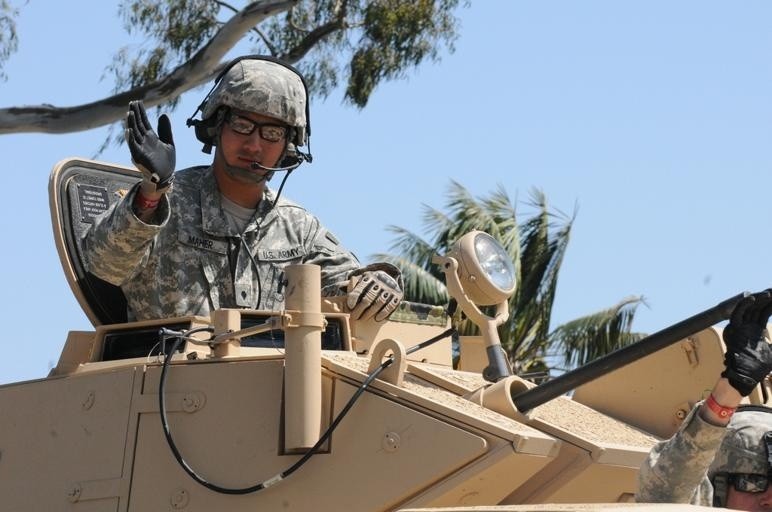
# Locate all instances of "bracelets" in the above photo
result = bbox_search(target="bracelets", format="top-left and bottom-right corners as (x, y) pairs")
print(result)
(138, 193), (162, 209)
(706, 393), (737, 420)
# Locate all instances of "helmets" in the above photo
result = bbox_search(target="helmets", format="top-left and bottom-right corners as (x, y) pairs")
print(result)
(706, 404), (772, 482)
(201, 59), (308, 147)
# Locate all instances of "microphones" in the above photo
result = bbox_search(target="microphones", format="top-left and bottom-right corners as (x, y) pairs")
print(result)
(250, 161), (303, 171)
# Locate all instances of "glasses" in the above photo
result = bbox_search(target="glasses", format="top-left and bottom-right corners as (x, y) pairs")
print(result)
(715, 472), (772, 494)
(223, 112), (290, 143)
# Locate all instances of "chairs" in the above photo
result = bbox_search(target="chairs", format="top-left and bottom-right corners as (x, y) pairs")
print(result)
(46, 154), (146, 334)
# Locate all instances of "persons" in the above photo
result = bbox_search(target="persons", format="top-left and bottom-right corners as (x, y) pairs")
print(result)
(632, 287), (772, 512)
(80, 54), (405, 323)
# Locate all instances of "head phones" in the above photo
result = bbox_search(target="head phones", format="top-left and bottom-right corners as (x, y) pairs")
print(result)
(185, 54), (312, 171)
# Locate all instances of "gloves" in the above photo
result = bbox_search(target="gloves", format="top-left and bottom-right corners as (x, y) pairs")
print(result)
(721, 287), (772, 397)
(345, 270), (404, 321)
(123, 98), (176, 200)
